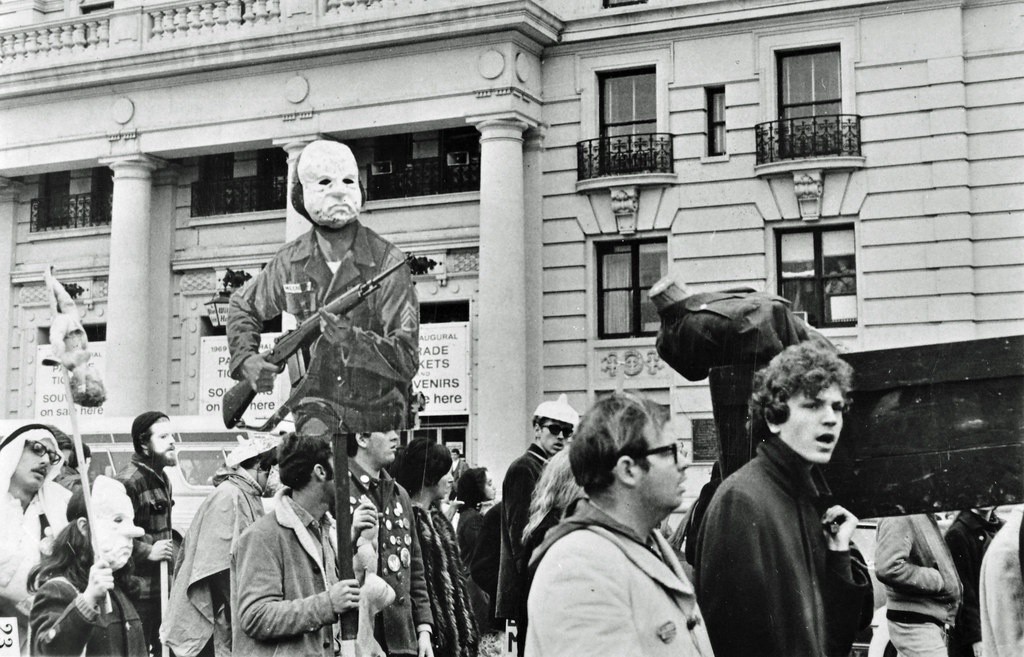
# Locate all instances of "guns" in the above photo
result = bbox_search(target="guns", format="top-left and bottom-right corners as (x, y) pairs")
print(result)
(221, 250), (417, 431)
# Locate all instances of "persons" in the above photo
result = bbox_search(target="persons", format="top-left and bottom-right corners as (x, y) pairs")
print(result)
(522, 396), (714, 657)
(692, 339), (875, 657)
(0, 139), (588, 657)
(870, 503), (1024, 657)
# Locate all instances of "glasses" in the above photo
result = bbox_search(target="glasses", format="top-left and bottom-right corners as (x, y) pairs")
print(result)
(632, 442), (687, 465)
(542, 424), (574, 438)
(26, 441), (62, 465)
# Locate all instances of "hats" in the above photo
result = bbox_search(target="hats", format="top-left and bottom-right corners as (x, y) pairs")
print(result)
(132, 411), (169, 442)
(533, 393), (579, 428)
